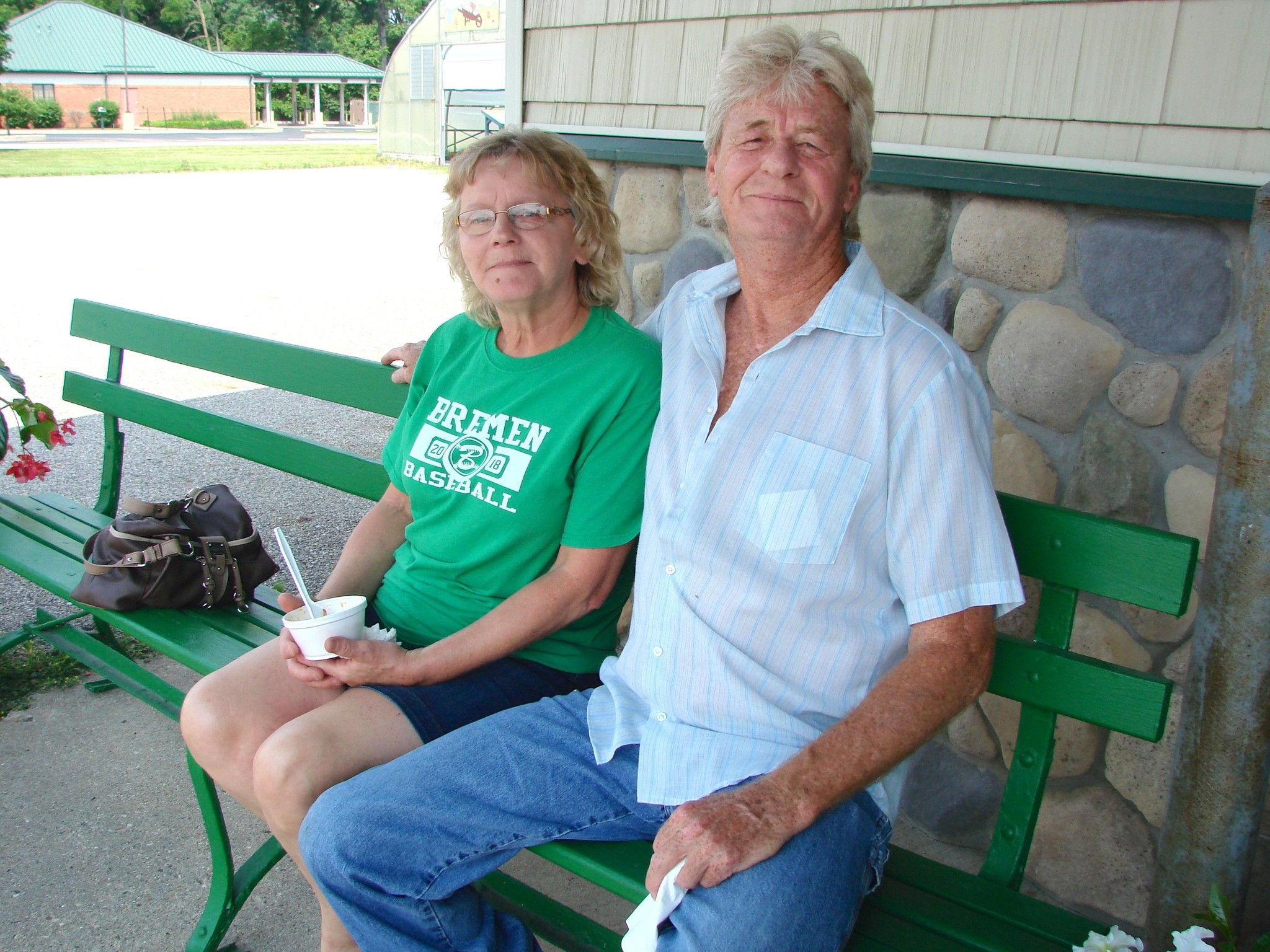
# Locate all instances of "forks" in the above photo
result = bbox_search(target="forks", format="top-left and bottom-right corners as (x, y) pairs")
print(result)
(274, 526), (328, 619)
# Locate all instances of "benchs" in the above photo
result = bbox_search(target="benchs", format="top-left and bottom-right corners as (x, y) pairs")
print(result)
(0, 300), (1200, 952)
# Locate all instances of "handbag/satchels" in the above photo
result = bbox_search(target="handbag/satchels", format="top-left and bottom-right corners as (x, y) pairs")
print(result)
(69, 483), (280, 615)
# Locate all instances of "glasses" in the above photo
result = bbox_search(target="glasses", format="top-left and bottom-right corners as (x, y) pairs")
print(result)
(455, 203), (571, 236)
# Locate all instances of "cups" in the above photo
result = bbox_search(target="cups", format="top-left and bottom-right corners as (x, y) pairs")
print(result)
(282, 594), (368, 660)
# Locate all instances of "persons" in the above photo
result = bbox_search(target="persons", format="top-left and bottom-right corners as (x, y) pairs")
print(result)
(179, 128), (661, 952)
(299, 22), (1025, 952)
